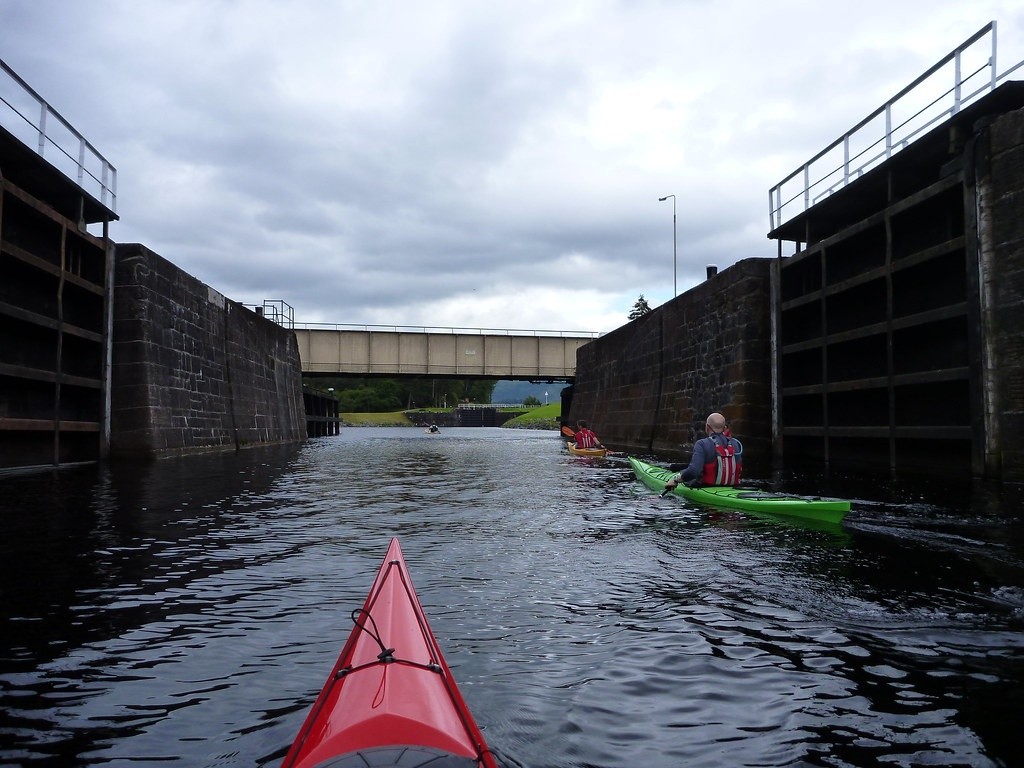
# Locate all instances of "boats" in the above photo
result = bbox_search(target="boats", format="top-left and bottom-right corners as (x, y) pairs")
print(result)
(424, 427), (441, 435)
(627, 454), (852, 526)
(567, 441), (608, 458)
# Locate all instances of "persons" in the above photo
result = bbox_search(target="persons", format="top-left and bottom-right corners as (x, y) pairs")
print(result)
(429, 421), (438, 433)
(673, 412), (744, 488)
(574, 420), (602, 449)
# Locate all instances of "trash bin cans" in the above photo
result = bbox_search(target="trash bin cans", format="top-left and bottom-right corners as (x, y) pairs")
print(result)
(706, 264), (717, 280)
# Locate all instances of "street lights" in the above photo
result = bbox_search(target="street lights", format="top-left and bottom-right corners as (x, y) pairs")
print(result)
(659, 194), (677, 298)
